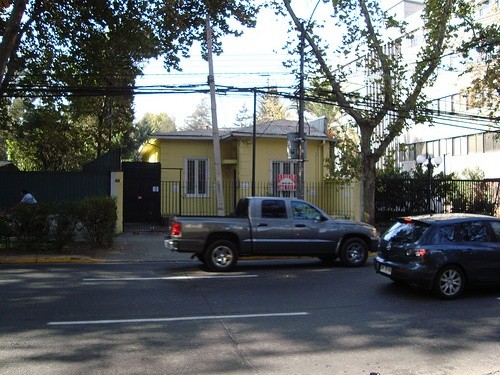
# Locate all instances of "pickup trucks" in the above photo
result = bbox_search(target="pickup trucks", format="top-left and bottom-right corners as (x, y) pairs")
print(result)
(163, 196), (381, 273)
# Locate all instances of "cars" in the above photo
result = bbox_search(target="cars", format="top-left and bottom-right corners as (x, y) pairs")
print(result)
(371, 211), (500, 301)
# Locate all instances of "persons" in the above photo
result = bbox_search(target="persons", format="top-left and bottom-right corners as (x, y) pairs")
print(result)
(16, 190), (39, 206)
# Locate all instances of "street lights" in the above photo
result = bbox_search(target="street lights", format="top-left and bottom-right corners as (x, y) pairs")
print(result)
(415, 154), (443, 214)
